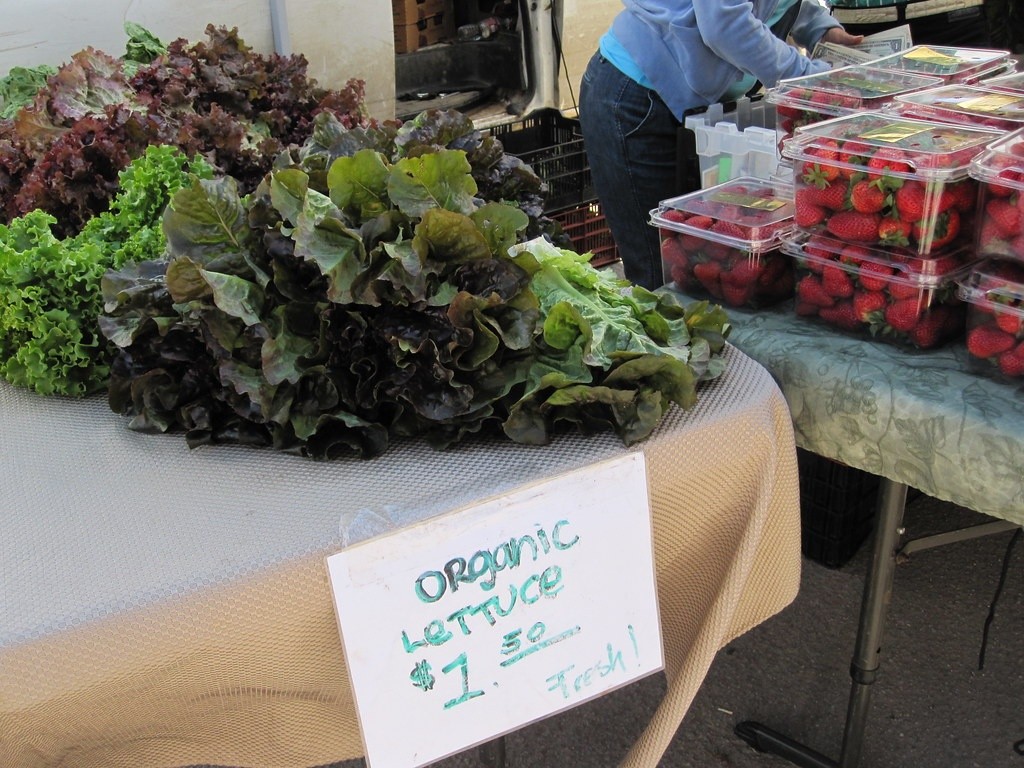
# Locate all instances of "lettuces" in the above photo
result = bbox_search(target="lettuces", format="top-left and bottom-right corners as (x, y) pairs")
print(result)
(0, 21), (403, 396)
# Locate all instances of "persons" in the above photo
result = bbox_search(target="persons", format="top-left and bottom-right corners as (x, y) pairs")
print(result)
(579, 0), (863, 292)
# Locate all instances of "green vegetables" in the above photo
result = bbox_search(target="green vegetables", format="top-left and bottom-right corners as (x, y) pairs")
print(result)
(98, 110), (731, 462)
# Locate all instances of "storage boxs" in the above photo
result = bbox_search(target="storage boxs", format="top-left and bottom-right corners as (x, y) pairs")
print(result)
(474, 105), (619, 267)
(797, 445), (879, 569)
(647, 44), (1024, 382)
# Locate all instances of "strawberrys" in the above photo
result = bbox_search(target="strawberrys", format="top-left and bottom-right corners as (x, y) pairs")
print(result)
(658, 47), (1024, 384)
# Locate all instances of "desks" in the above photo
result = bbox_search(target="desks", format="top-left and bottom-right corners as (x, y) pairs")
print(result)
(652, 282), (1024, 768)
(0, 330), (801, 768)
